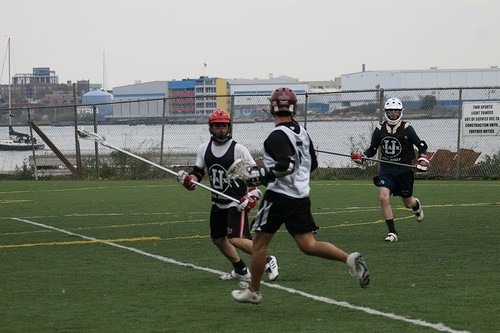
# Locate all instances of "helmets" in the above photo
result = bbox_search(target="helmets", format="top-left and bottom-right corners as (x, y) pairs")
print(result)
(270, 88), (297, 117)
(384, 98), (403, 125)
(208, 110), (232, 143)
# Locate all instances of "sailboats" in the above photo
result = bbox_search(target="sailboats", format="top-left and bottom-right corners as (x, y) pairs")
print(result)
(0, 37), (45, 151)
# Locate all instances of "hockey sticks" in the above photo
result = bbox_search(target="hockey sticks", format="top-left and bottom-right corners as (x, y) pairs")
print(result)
(78, 128), (242, 204)
(315, 149), (417, 170)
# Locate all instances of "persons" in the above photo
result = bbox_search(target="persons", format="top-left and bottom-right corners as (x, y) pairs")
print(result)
(178, 109), (280, 282)
(352, 99), (429, 242)
(231, 87), (369, 304)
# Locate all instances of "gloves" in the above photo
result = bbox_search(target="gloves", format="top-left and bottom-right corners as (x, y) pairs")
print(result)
(177, 175), (196, 191)
(246, 166), (275, 187)
(415, 156), (430, 172)
(239, 188), (257, 212)
(351, 152), (364, 164)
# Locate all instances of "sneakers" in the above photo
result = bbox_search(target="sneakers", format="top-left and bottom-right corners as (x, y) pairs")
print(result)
(347, 252), (369, 288)
(384, 232), (398, 242)
(264, 255), (280, 281)
(412, 198), (424, 222)
(219, 267), (252, 281)
(231, 288), (262, 305)
